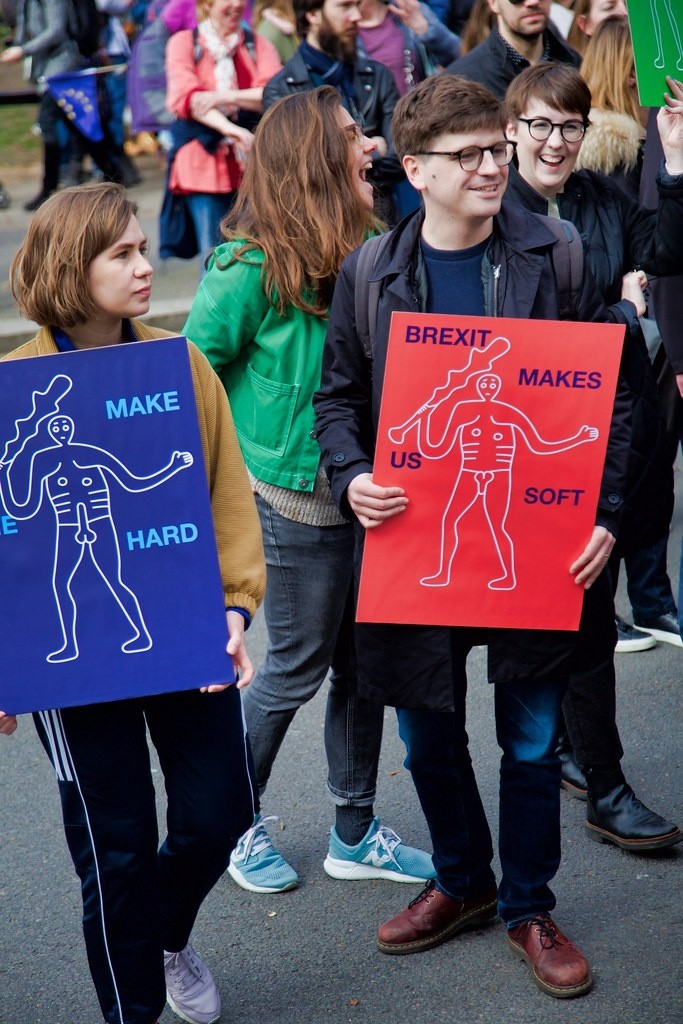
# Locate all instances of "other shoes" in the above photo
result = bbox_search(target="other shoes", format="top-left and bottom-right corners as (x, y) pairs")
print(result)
(24, 192), (48, 210)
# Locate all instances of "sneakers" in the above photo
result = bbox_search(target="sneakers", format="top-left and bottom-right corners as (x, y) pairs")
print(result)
(227, 814), (298, 893)
(163, 942), (222, 1024)
(614, 614), (657, 652)
(323, 815), (438, 884)
(632, 610), (683, 648)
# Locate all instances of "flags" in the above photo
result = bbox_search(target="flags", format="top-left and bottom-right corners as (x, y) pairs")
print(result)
(40, 69), (104, 141)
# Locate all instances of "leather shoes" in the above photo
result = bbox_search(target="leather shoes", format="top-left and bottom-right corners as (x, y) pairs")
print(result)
(585, 777), (683, 850)
(508, 912), (593, 998)
(376, 878), (498, 954)
(558, 752), (589, 800)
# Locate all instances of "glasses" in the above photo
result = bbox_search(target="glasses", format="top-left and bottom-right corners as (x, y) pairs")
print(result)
(417, 141), (517, 172)
(509, 0), (525, 4)
(518, 118), (589, 143)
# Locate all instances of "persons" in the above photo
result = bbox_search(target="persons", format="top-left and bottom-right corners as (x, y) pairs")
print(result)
(311, 73), (631, 998)
(175, 85), (441, 894)
(501, 61), (683, 852)
(0, 183), (267, 1024)
(0, 0), (683, 654)
